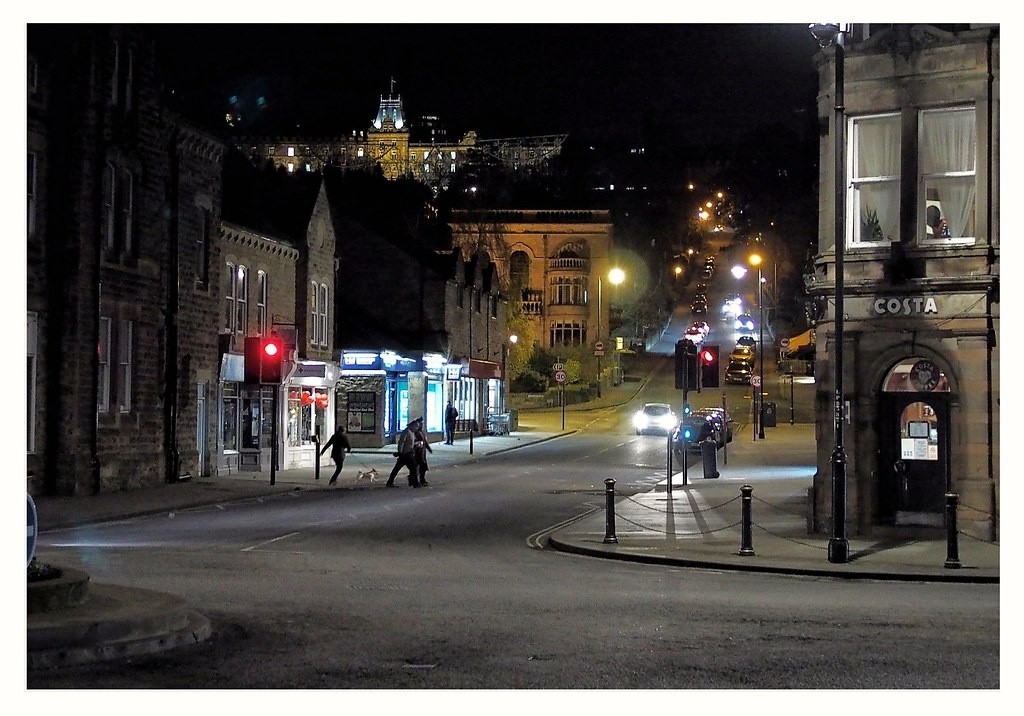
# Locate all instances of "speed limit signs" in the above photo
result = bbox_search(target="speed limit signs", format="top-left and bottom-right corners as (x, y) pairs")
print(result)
(555, 372), (567, 382)
(752, 375), (762, 386)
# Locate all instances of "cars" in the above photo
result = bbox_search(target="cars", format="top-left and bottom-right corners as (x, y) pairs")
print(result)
(672, 407), (734, 456)
(636, 402), (675, 437)
(735, 336), (757, 351)
(684, 321), (710, 346)
(722, 294), (743, 322)
(729, 346), (755, 369)
(689, 256), (715, 315)
(725, 361), (753, 385)
(735, 315), (754, 333)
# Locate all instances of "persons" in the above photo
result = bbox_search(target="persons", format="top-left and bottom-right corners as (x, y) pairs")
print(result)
(444, 400), (459, 445)
(386, 417), (432, 489)
(629, 341), (637, 350)
(927, 206), (952, 239)
(320, 425), (351, 486)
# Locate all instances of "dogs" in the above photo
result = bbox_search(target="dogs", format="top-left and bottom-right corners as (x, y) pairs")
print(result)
(354, 468), (379, 482)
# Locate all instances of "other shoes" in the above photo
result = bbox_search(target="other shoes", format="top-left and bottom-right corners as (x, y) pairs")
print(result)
(444, 442), (450, 444)
(408, 481), (420, 488)
(386, 482), (399, 488)
(451, 442), (453, 445)
(329, 481), (336, 485)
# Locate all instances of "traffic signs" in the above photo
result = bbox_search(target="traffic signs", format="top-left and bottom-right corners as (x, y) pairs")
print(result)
(553, 363), (563, 370)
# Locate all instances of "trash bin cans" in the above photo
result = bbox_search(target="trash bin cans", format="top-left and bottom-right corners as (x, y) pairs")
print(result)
(763, 402), (776, 427)
(509, 409), (518, 431)
(699, 440), (720, 479)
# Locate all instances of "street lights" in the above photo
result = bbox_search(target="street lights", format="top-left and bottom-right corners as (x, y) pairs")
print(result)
(597, 268), (625, 398)
(808, 23), (850, 564)
(748, 254), (764, 440)
(674, 266), (681, 309)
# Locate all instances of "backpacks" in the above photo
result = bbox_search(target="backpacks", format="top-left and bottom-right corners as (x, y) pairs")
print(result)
(449, 407), (458, 418)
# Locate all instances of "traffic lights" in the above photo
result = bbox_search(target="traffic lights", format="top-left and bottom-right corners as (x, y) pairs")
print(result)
(684, 426), (693, 440)
(701, 345), (719, 388)
(684, 404), (690, 414)
(259, 336), (283, 386)
